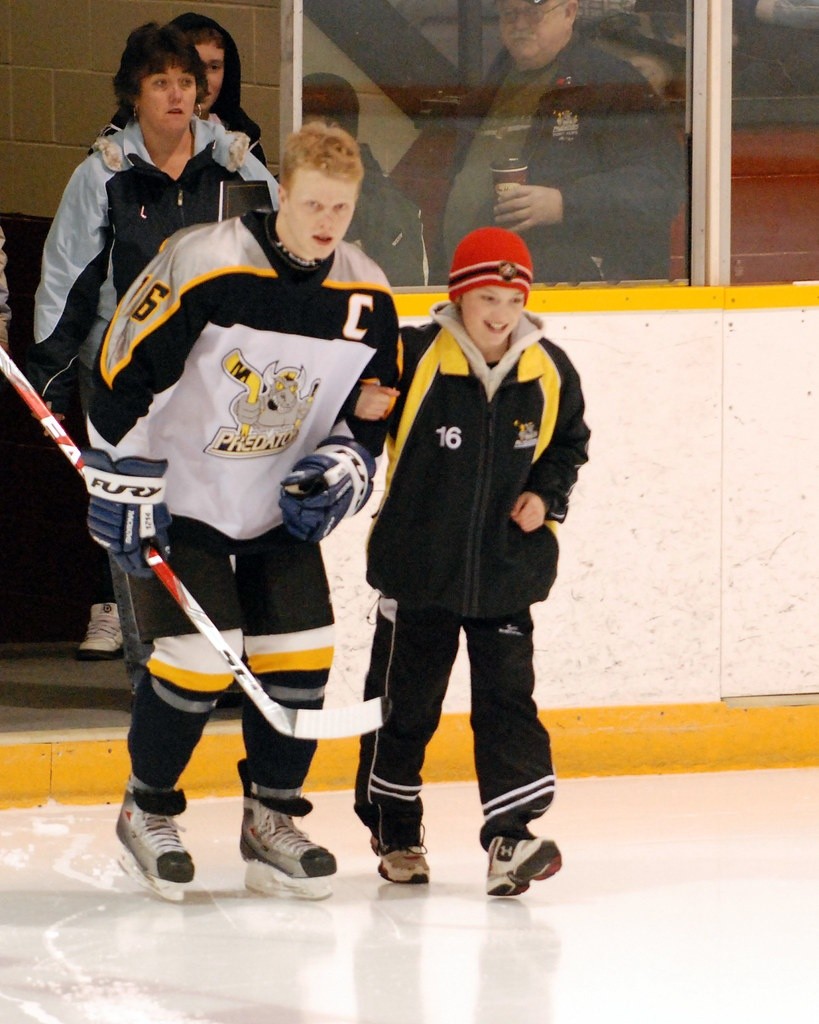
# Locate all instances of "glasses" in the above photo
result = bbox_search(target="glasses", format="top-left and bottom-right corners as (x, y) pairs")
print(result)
(499, 2), (562, 25)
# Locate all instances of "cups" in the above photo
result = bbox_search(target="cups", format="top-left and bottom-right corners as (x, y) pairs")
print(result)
(490, 158), (528, 226)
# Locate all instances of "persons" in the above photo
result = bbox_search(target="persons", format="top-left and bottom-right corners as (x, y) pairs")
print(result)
(434, 0), (686, 282)
(346, 225), (592, 898)
(34, 10), (290, 655)
(300, 71), (431, 288)
(84, 121), (403, 902)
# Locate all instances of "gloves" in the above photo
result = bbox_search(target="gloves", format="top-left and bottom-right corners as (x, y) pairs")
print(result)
(280, 437), (376, 542)
(81, 448), (169, 578)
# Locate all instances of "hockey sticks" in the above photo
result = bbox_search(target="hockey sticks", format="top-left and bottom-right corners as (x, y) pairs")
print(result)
(0, 348), (390, 743)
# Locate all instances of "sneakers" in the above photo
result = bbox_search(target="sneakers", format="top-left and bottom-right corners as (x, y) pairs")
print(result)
(241, 796), (337, 901)
(115, 775), (195, 902)
(80, 603), (124, 661)
(370, 823), (430, 884)
(486, 835), (561, 896)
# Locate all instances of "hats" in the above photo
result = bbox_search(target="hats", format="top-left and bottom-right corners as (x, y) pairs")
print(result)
(448, 227), (533, 306)
